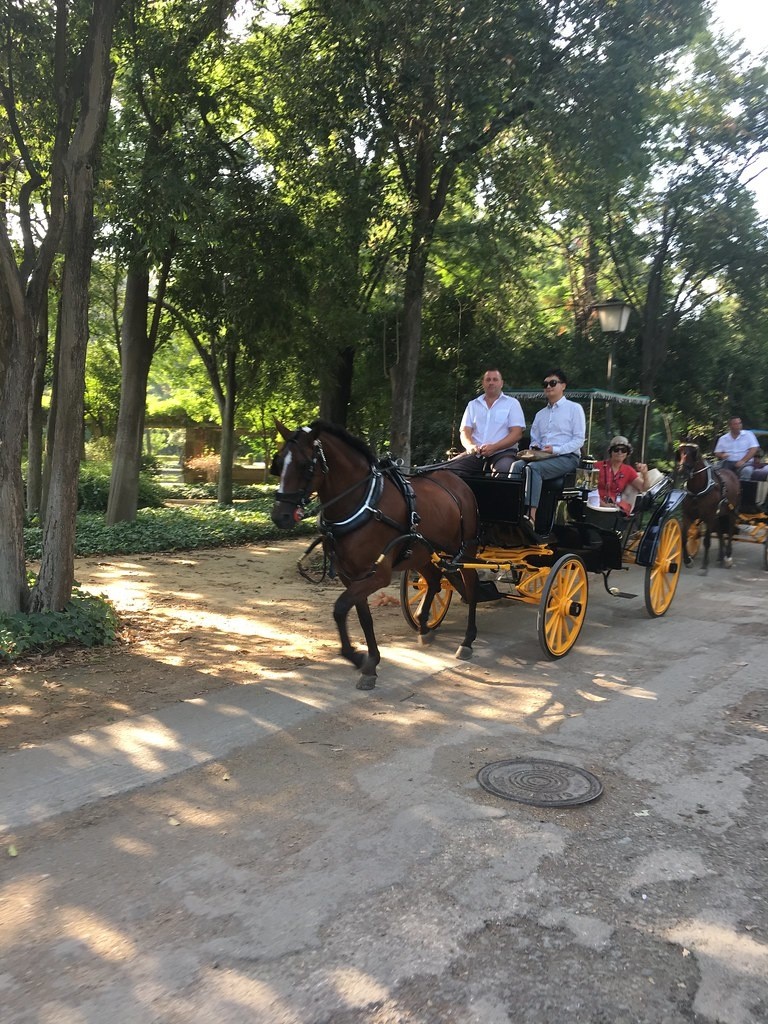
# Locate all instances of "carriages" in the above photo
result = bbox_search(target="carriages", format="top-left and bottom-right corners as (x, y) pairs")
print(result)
(269, 383), (691, 692)
(675, 428), (768, 577)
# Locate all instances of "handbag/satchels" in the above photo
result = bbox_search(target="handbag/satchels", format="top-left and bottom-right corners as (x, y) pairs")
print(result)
(516, 449), (557, 460)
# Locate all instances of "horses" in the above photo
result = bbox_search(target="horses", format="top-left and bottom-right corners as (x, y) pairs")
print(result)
(269, 414), (482, 690)
(675, 439), (742, 576)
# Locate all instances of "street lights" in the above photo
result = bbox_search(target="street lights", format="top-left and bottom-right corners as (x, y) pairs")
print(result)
(586, 290), (633, 456)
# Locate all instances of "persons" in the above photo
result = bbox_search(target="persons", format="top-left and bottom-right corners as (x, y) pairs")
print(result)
(594, 435), (651, 515)
(713, 416), (760, 481)
(448, 367), (586, 532)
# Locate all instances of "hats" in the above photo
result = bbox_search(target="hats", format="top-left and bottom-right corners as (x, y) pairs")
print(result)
(608, 435), (633, 457)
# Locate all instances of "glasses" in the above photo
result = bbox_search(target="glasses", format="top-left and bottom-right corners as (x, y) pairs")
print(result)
(612, 448), (628, 453)
(542, 380), (564, 388)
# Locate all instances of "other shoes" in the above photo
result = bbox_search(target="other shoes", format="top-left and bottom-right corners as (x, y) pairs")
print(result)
(523, 515), (536, 532)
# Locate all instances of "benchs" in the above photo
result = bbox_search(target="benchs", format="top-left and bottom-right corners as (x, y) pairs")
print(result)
(516, 438), (577, 533)
